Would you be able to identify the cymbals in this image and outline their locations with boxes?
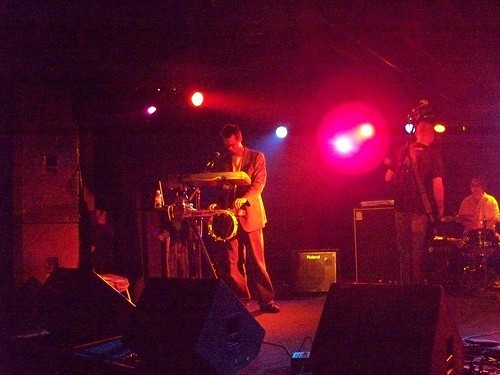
[207,209,241,242]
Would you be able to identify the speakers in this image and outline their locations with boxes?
[36,267,136,344]
[121,277,265,374]
[297,253,338,292]
[353,209,404,283]
[309,280,440,373]
[0,135,77,222]
[0,222,78,288]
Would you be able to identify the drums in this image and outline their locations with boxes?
[467,228,498,257]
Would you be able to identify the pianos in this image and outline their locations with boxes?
[167,171,252,277]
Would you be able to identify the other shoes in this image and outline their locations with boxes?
[490,278,500,289]
[259,302,280,312]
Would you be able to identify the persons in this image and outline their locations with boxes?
[207,124,280,313]
[458,174,500,288]
[384,121,445,281]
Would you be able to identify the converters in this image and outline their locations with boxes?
[290,352,311,370]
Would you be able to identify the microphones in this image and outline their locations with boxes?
[411,100,429,112]
[206,151,220,167]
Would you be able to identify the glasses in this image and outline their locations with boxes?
[223,143,238,149]
